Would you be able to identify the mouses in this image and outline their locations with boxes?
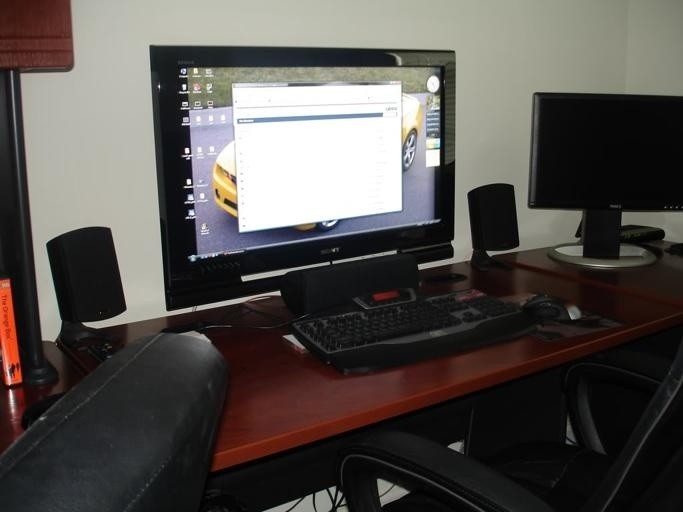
[526,293,581,323]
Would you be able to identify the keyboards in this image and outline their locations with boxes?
[292,287,537,374]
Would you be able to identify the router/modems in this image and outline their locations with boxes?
[620,224,666,245]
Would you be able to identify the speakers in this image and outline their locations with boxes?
[468,184,519,272]
[46,227,126,364]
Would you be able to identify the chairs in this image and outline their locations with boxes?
[0,328,230,512]
[336,345,682,512]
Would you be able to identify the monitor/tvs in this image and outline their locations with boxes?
[149,45,455,319]
[527,93,683,269]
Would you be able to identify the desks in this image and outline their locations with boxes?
[2,232,681,467]
[490,226,682,310]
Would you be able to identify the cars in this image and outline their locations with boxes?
[209,90,423,235]
